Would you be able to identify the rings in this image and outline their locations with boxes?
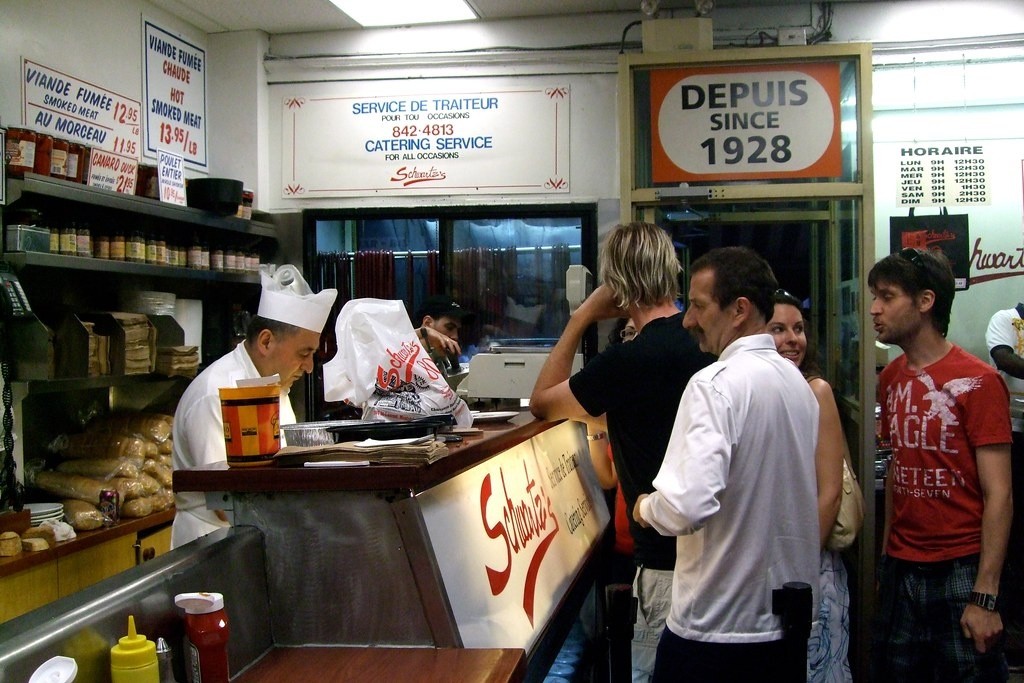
[446,339,449,342]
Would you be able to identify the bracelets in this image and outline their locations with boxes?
[586,431,606,441]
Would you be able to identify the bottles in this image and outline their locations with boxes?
[110,616,174,683]
[174,592,229,683]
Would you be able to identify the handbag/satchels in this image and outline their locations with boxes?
[808,377,865,550]
[320,297,474,434]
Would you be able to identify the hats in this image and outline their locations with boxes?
[257,264,338,332]
[419,295,472,318]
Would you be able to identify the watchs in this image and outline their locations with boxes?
[967,590,998,613]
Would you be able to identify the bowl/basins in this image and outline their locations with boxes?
[281,419,385,448]
[187,178,243,216]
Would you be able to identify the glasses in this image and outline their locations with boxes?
[900,248,927,283]
[774,288,794,296]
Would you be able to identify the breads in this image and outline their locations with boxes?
[0,409,175,558]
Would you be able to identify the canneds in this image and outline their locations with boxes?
[4,124,92,185]
[134,163,160,200]
[236,190,254,221]
[45,221,260,272]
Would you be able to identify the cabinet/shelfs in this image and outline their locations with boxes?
[0,171,281,494]
[140,526,172,564]
[0,530,139,625]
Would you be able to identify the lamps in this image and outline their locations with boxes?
[641,0,713,52]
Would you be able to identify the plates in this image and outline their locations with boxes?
[9,503,66,527]
[472,411,519,422]
[325,420,445,443]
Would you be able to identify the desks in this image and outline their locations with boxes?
[228,644,529,683]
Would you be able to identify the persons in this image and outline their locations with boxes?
[587,316,638,683]
[986,303,1023,672]
[481,296,545,339]
[171,264,338,552]
[766,287,855,683]
[868,248,1012,683]
[414,296,472,365]
[632,248,820,683]
[530,220,719,683]
